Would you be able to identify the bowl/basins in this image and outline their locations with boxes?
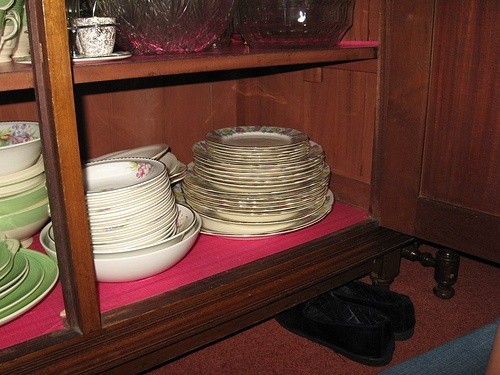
[90,0,237,55]
[234,1,355,51]
[38,202,203,283]
[0,182,49,251]
[0,122,41,174]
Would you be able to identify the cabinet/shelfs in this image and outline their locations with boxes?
[0,0,500,375]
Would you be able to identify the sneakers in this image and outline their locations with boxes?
[332,280,415,341]
[275,293,394,366]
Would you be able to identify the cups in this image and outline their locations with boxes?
[66,1,116,56]
[0,0,30,63]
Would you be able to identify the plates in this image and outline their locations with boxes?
[79,127,334,252]
[0,154,47,200]
[71,51,133,64]
[0,234,59,328]
